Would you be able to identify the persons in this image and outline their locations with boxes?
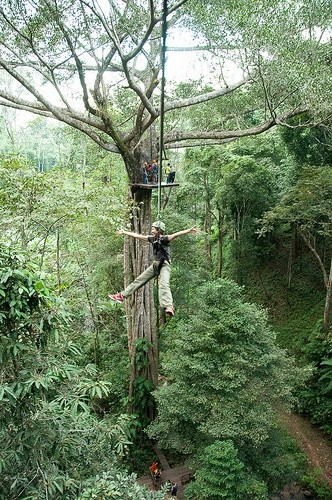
[152,469,178,498]
[141,159,175,185]
[107,221,201,317]
